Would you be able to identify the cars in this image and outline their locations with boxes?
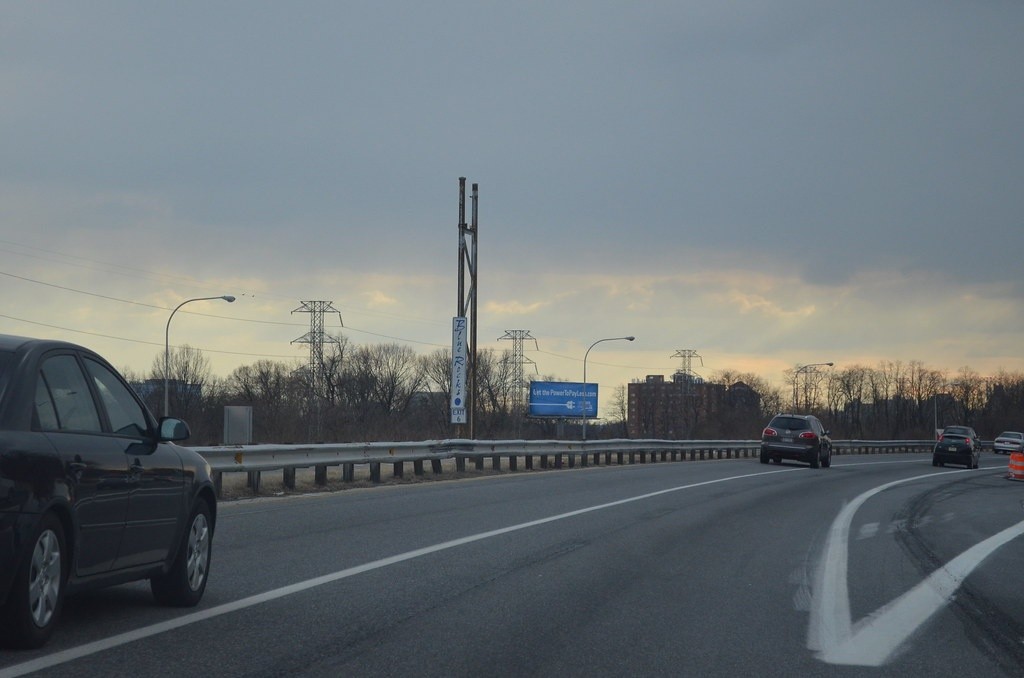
[994,430,1024,454]
[0,334,217,652]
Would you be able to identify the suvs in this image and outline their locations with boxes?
[933,433,976,469]
[944,425,981,454]
[761,412,832,469]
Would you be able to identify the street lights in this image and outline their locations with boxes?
[164,296,236,416]
[935,383,960,441]
[792,363,833,413]
[583,337,635,440]
